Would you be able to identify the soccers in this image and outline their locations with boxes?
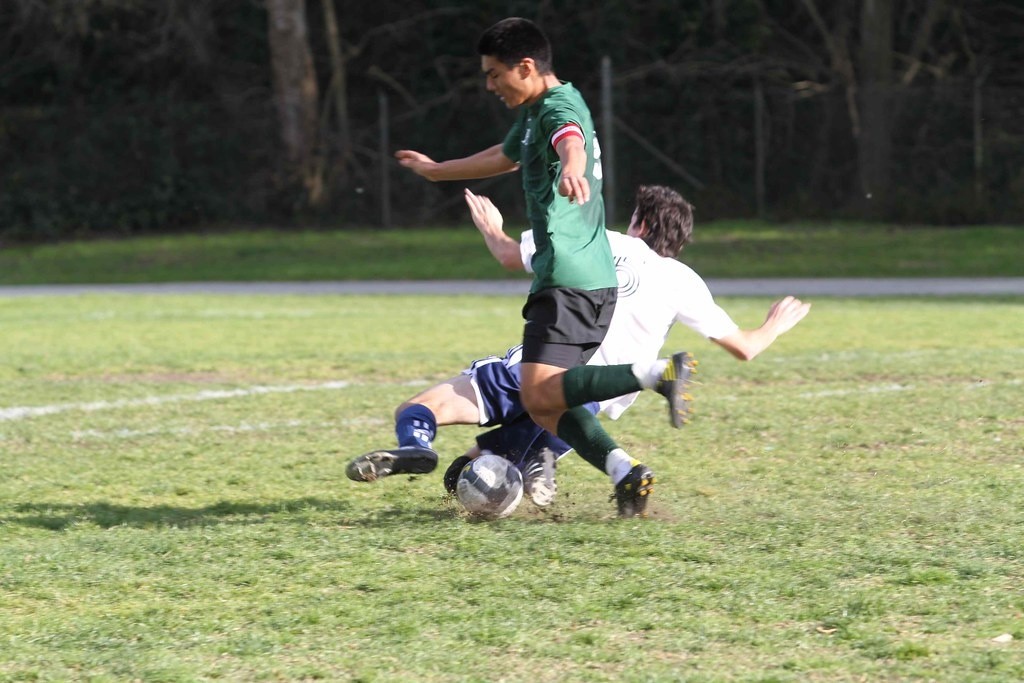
[456,453,525,522]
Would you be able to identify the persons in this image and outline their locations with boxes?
[344,187,811,505]
[394,17,700,518]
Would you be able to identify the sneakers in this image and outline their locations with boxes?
[608,460,656,518]
[346,445,438,483]
[522,447,558,506]
[654,352,699,430]
